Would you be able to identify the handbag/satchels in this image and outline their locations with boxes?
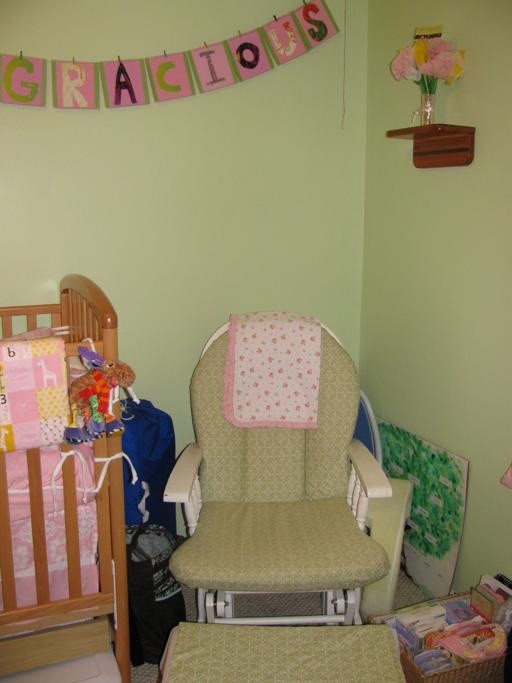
[120,397,177,540]
[107,521,189,667]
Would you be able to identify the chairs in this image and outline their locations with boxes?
[162,312,393,629]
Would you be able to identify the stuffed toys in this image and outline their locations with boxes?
[64,346,136,444]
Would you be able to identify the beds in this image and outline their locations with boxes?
[1,271,132,683]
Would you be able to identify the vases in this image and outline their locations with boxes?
[421,92,435,126]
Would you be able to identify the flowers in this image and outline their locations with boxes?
[390,36,466,94]
